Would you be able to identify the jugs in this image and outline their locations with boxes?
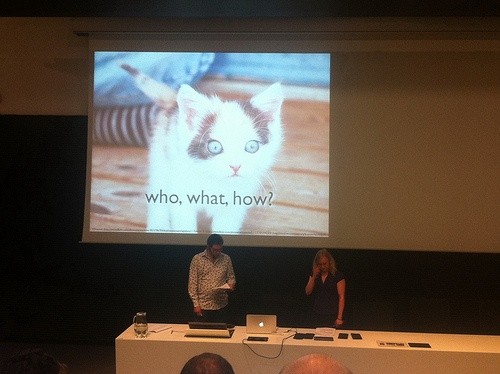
[132,312,149,337]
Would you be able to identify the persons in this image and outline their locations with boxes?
[179,353,234,374]
[188,234,236,323]
[304,249,345,329]
[278,353,352,374]
[1,349,69,374]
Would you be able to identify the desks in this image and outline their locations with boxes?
[115,322,500,374]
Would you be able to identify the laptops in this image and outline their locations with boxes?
[246,314,279,334]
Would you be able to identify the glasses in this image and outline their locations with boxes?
[213,249,223,252]
